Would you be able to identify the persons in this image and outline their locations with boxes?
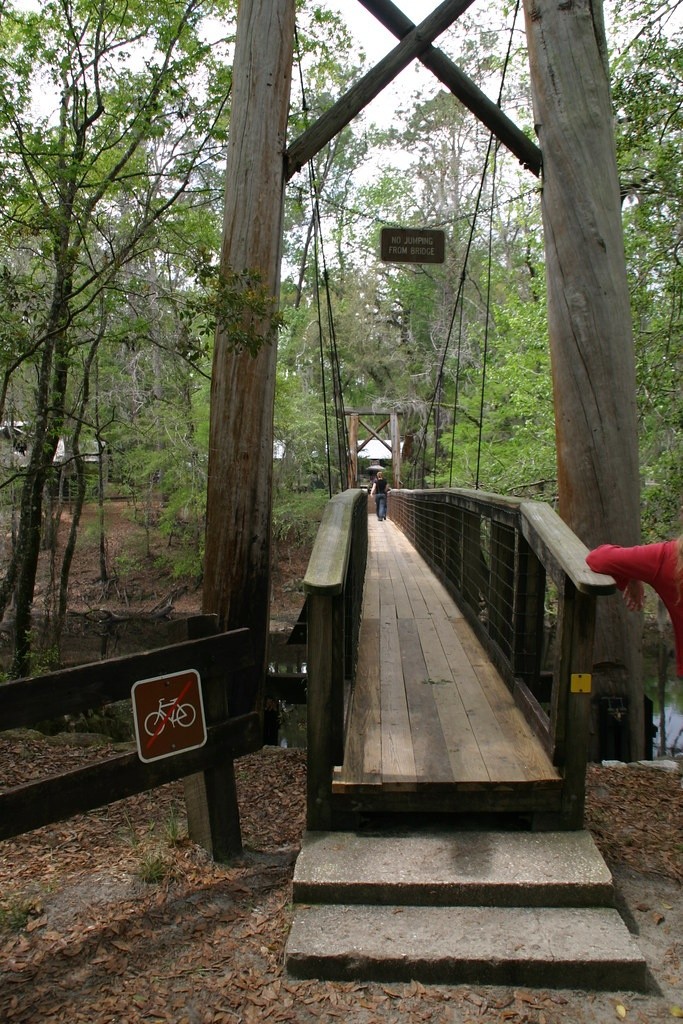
[370,471,388,521]
[585,534,683,678]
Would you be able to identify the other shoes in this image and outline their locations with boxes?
[378,517,383,521]
[383,515,387,520]
